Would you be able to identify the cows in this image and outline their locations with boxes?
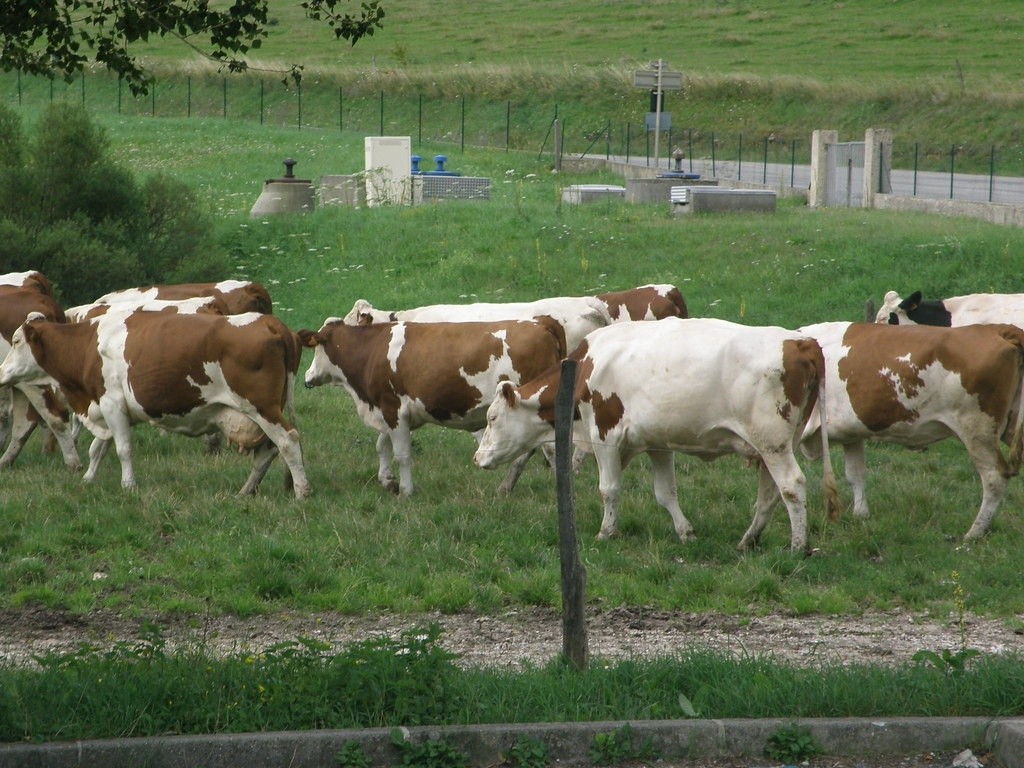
[1,268,1024,559]
